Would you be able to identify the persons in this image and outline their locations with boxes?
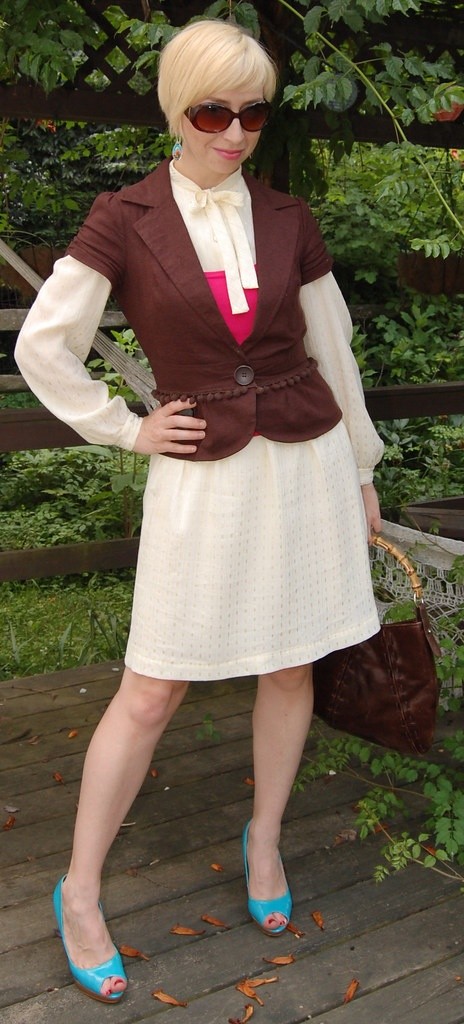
[14,20,385,1003]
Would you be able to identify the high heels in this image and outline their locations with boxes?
[53,875,127,1004]
[241,819,292,937]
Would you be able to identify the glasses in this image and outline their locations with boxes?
[187,97,275,134]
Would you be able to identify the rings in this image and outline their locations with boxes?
[373,528,382,534]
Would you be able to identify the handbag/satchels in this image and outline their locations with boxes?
[311,533,443,754]
